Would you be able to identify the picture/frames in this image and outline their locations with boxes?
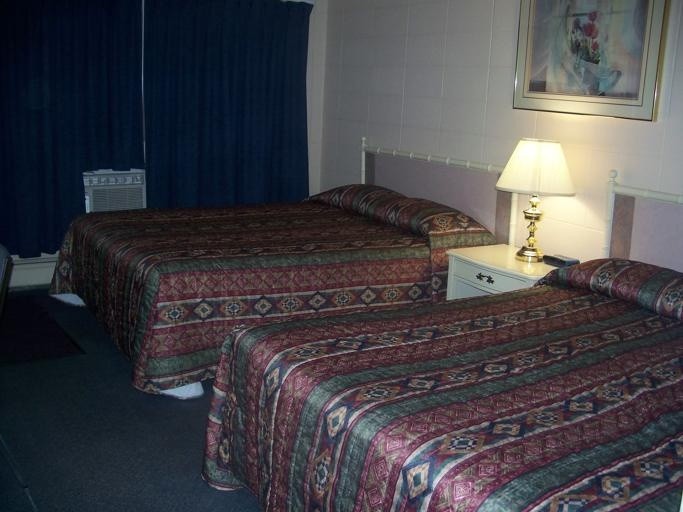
[511,0,672,124]
